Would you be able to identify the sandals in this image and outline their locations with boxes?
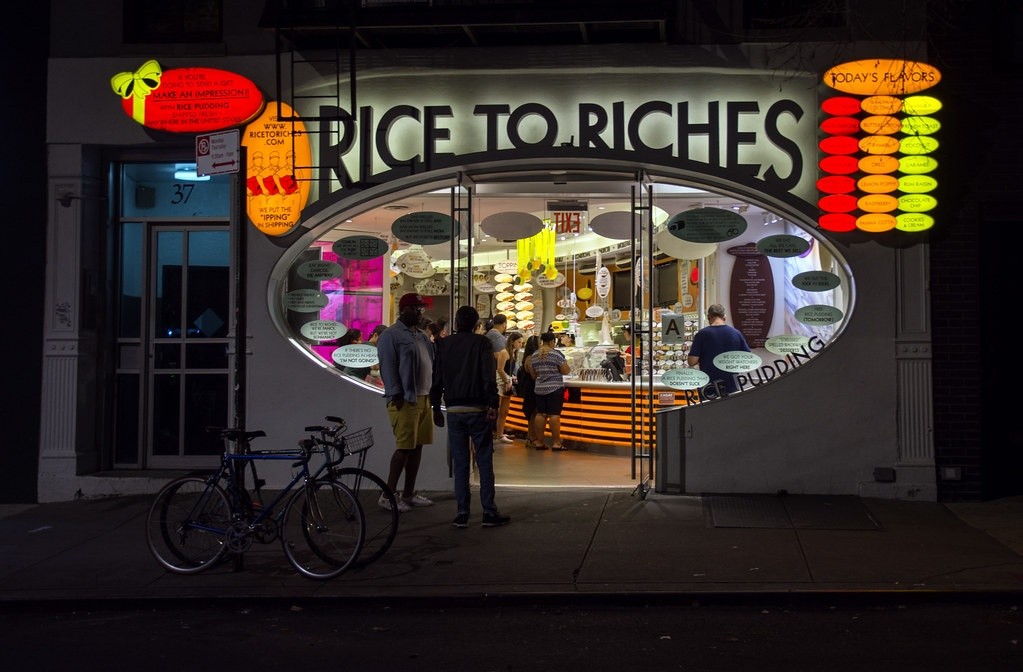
[525,438,537,446]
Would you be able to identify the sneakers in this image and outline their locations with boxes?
[378,491,413,513]
[400,493,433,507]
[481,513,511,526]
[452,513,469,527]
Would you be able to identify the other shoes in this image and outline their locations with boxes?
[503,434,515,438]
[497,436,513,443]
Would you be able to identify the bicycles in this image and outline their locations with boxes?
[145,413,401,580]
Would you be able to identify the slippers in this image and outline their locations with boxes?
[536,444,549,450]
[552,445,568,451]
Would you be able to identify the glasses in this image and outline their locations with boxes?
[415,307,425,314]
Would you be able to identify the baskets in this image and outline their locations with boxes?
[338,427,373,457]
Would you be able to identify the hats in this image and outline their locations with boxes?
[621,323,640,330]
[399,293,429,307]
[560,333,574,339]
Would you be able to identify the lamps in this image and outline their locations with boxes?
[764,213,783,226]
[174,164,210,181]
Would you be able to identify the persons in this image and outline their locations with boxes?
[429,306,511,528]
[620,321,641,382]
[686,304,752,402]
[333,314,575,452]
[377,292,435,513]
